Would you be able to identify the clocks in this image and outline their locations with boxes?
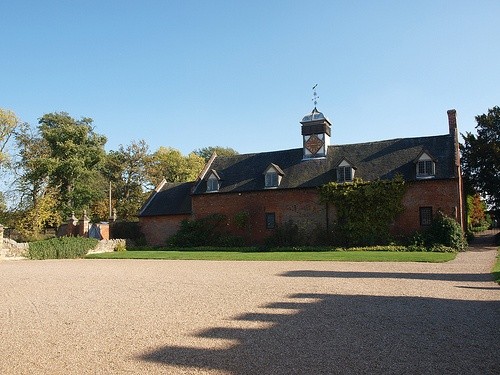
[304,134,324,157]
